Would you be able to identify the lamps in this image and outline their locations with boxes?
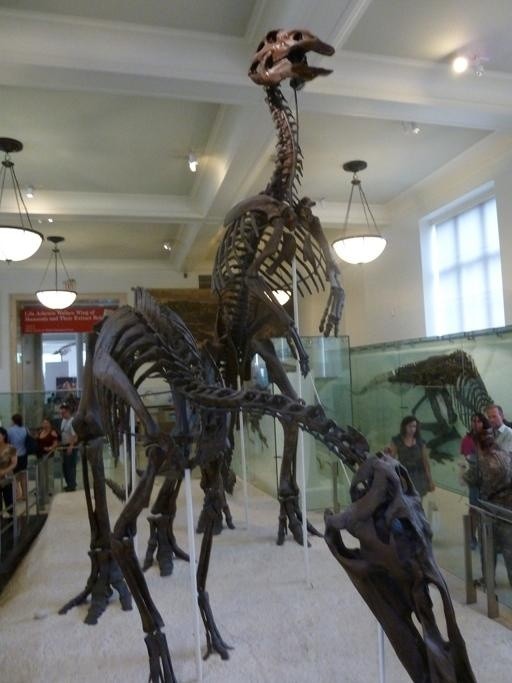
[330,159,388,266]
[261,267,292,306]
[1,136,45,263]
[26,184,33,198]
[185,150,199,174]
[451,48,488,80]
[32,236,79,312]
[400,119,423,136]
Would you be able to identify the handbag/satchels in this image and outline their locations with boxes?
[426,499,442,533]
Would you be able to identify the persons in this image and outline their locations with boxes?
[475,430,512,583]
[0,426,17,525]
[486,402,511,453]
[59,405,78,491]
[34,417,59,495]
[458,412,489,548]
[7,414,30,499]
[391,415,435,503]
